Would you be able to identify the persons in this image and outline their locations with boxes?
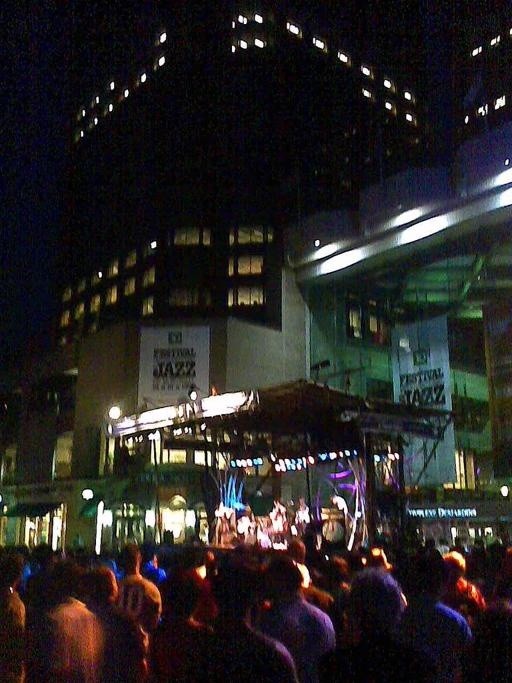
[236,503,261,546]
[212,499,236,544]
[293,497,311,535]
[2,519,510,683]
[268,498,288,546]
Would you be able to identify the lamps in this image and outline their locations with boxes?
[109,405,126,422]
[188,382,204,414]
[310,359,330,388]
